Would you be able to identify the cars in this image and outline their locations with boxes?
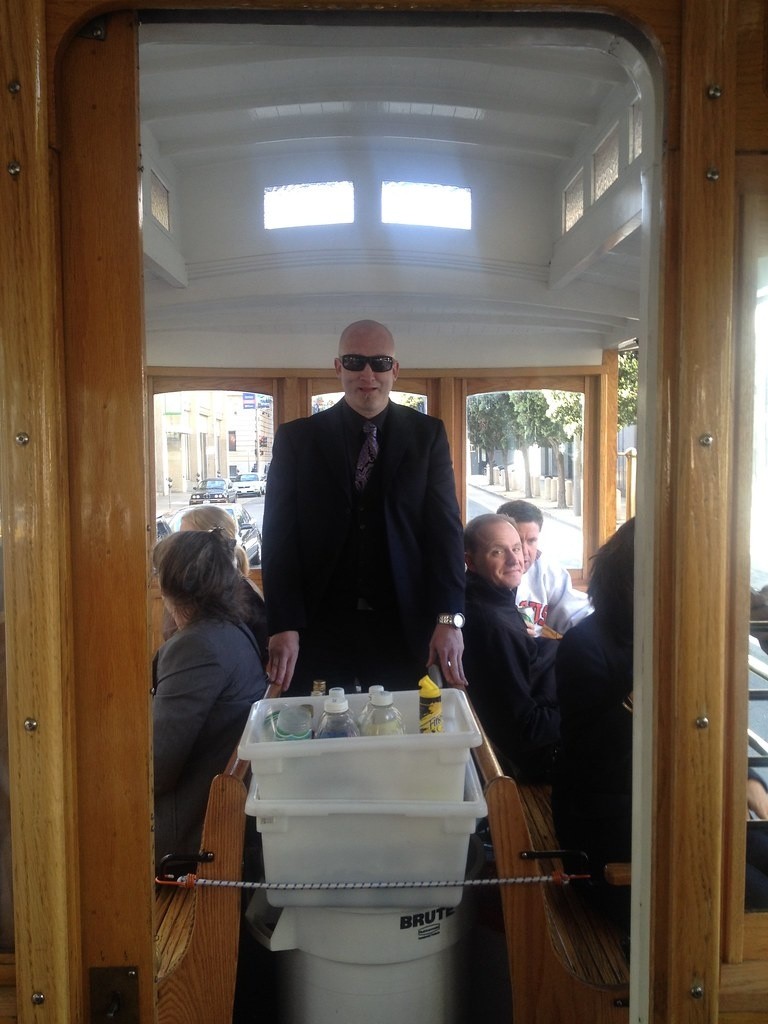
[157,502,262,568]
[189,472,268,505]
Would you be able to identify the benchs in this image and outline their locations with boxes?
[88,682,281,1024]
[444,678,631,1024]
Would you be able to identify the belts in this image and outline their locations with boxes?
[323,598,396,610]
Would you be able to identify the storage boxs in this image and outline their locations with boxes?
[237,686,488,909]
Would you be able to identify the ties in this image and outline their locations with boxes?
[354,421,380,497]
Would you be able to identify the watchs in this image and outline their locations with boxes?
[438,613,465,628]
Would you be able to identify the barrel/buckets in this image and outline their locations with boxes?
[242,835,487,1024]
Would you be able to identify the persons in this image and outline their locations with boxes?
[152,507,266,874]
[464,502,768,915]
[261,320,468,691]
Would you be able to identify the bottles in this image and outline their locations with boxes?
[268,679,407,743]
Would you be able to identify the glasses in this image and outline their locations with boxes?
[339,355,395,372]
